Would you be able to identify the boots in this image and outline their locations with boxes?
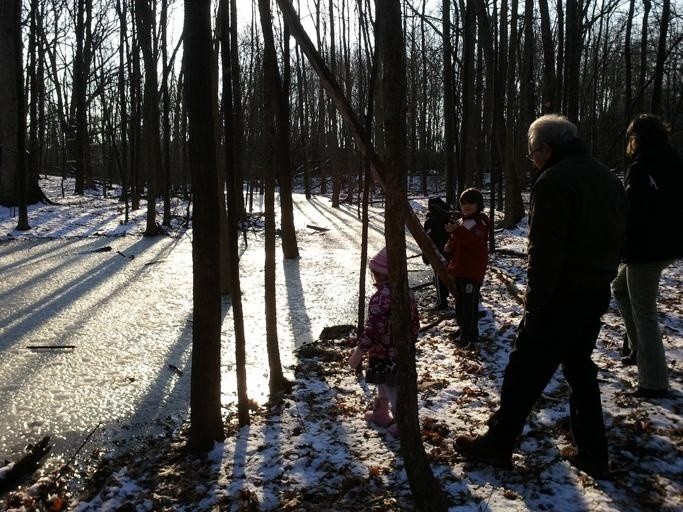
[365,397,400,437]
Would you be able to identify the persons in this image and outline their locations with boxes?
[348,246,421,429]
[613,115,683,400]
[444,187,496,347]
[456,113,630,468]
[422,197,460,312]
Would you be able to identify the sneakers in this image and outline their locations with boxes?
[621,351,636,366]
[450,328,479,346]
[453,434,512,465]
[623,387,668,399]
[429,301,448,311]
[560,446,605,473]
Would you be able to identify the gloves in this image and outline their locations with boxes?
[348,346,364,369]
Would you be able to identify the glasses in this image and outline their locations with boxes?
[525,146,543,161]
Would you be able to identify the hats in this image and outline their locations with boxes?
[369,247,388,274]
